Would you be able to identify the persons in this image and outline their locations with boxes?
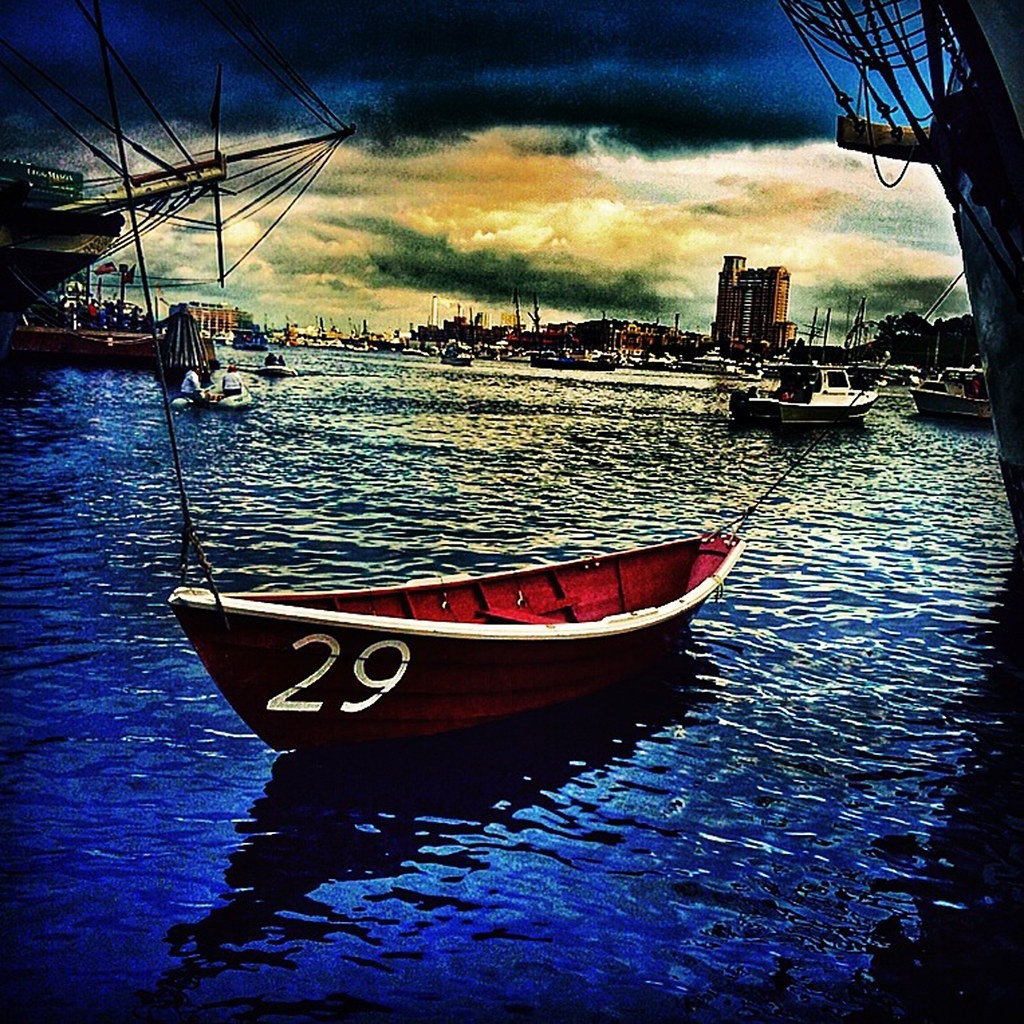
[180,351,289,408]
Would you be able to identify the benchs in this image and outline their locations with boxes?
[483,608,562,625]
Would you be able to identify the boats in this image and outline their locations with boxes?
[212,333,235,346]
[291,352,325,375]
[730,363,881,425]
[232,329,268,352]
[170,382,253,411]
[440,342,475,366]
[909,367,992,419]
[168,532,747,750]
[402,348,429,358]
[530,354,615,371]
[265,365,298,375]
[210,369,265,392]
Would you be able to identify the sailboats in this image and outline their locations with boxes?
[1,0,355,359]
